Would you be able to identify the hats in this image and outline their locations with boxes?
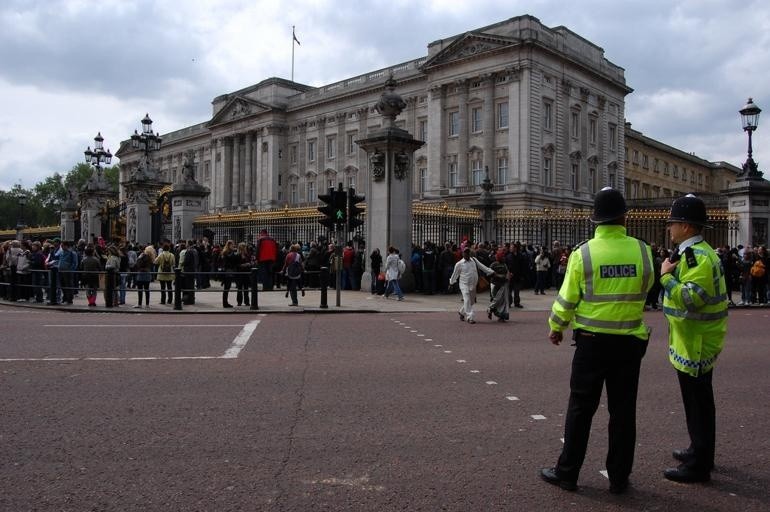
[664,193,713,230]
[588,187,629,224]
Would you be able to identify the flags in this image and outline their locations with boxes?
[293,31,300,46]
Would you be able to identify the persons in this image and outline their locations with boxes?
[370,248,382,293]
[506,244,523,308]
[487,251,513,323]
[542,188,655,494]
[382,246,405,301]
[2,230,366,306]
[448,247,496,324]
[460,240,477,303]
[636,237,769,312]
[659,194,728,484]
[410,236,577,295]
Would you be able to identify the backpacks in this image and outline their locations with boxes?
[162,253,171,272]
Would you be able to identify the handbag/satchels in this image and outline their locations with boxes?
[288,262,301,277]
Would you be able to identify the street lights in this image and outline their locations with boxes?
[735,98,767,183]
[80,131,112,191]
[127,113,162,182]
[15,192,27,226]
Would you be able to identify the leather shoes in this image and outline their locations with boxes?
[672,449,692,461]
[664,467,710,483]
[540,467,577,489]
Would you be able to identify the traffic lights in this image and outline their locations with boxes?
[316,187,335,231]
[332,191,347,225]
[346,187,366,233]
